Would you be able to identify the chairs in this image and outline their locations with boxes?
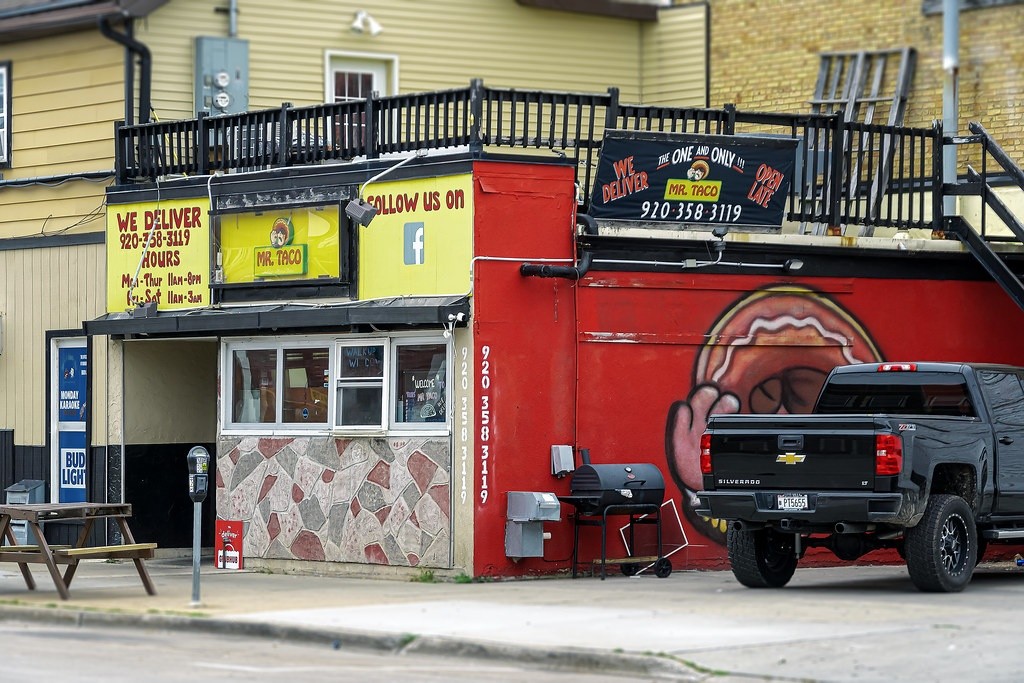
[923,396,970,416]
[843,395,885,413]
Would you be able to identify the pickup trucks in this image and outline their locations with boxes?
[695,361,1024,594]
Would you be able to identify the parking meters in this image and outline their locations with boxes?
[187,446,210,601]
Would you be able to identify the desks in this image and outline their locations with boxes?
[0,502,157,600]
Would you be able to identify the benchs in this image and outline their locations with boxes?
[57,543,157,555]
[0,544,72,551]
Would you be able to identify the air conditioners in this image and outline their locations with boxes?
[229,122,280,174]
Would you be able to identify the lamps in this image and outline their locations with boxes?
[713,226,730,252]
[129,177,160,318]
[785,259,803,271]
[350,10,367,35]
[365,16,384,37]
[344,148,428,228]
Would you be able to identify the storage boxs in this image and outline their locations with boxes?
[270,367,308,389]
